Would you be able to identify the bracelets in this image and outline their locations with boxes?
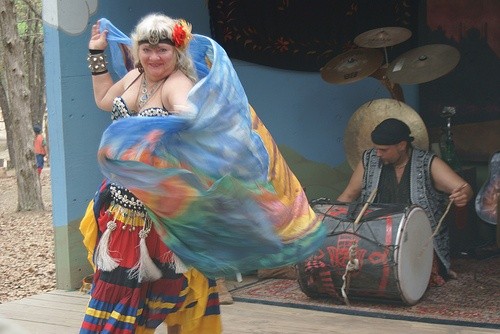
[89,49,109,75]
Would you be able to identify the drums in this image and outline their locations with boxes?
[295,196,434,306]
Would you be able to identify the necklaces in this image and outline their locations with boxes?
[396,166,405,171]
[134,71,167,112]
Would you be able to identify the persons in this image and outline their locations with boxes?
[336,117,473,283]
[79,12,328,334]
[33,126,46,176]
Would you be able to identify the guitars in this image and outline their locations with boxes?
[475,152,500,224]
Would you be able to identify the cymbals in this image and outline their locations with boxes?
[354,26,411,47]
[320,48,385,83]
[343,99,428,173]
[387,43,460,85]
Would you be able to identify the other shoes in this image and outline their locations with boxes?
[215,286,233,304]
[429,271,445,287]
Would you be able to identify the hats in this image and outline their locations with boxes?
[371,118,411,145]
